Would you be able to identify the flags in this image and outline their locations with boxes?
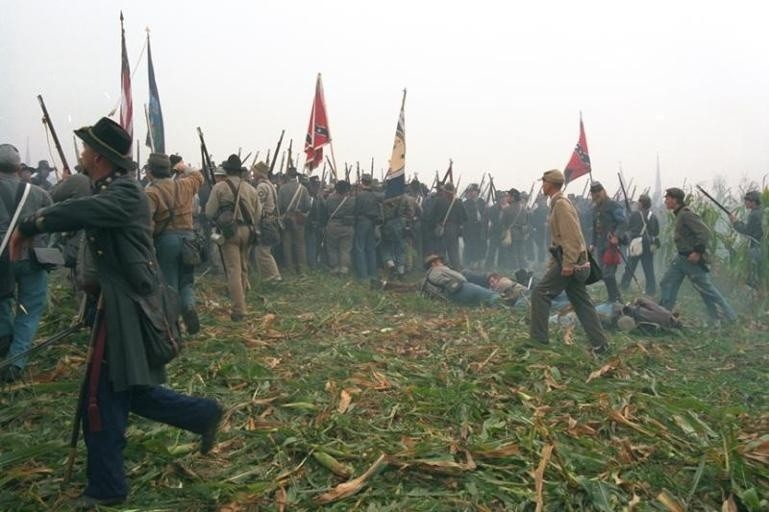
[144,39,165,155]
[118,34,134,140]
[304,75,332,171]
[562,122,591,183]
[382,100,406,199]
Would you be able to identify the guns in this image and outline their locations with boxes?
[607,233,646,297]
[527,173,651,209]
[415,173,423,198]
[135,139,142,181]
[695,184,737,220]
[430,159,497,205]
[38,94,73,177]
[63,288,104,482]
[197,127,376,189]
[72,134,81,162]
[212,221,226,274]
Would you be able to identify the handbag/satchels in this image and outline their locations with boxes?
[499,227,513,249]
[445,279,463,294]
[259,212,286,246]
[182,233,213,267]
[433,223,444,237]
[626,236,642,259]
[587,249,603,284]
[29,244,66,272]
[214,211,238,238]
[136,271,186,368]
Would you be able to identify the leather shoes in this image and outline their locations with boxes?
[4,365,20,383]
[180,300,201,336]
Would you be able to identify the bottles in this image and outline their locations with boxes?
[210,232,225,245]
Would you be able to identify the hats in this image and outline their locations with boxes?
[169,154,182,166]
[36,158,56,173]
[250,160,269,180]
[433,181,530,200]
[0,145,23,170]
[536,169,564,186]
[286,167,372,194]
[663,188,685,198]
[744,190,761,203]
[424,252,443,266]
[148,152,172,169]
[75,116,139,170]
[220,154,247,172]
[590,180,603,193]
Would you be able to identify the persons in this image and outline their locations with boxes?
[276,165,550,289]
[659,187,738,321]
[1,143,93,382]
[422,257,569,308]
[728,191,764,292]
[527,169,608,351]
[9,116,224,506]
[567,182,661,303]
[144,152,284,338]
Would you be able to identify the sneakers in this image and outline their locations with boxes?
[516,340,548,353]
[69,493,126,509]
[198,402,234,456]
